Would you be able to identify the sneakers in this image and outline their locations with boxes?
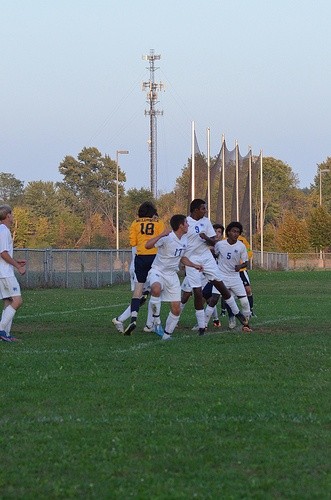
[221,307,227,317]
[112,317,124,333]
[212,319,222,327]
[0,330,16,342]
[242,317,251,332]
[228,316,236,328]
[123,321,137,336]
[192,323,208,330]
[251,309,257,318]
[144,324,155,332]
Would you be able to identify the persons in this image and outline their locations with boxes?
[112,199,257,341]
[0,206,28,342]
